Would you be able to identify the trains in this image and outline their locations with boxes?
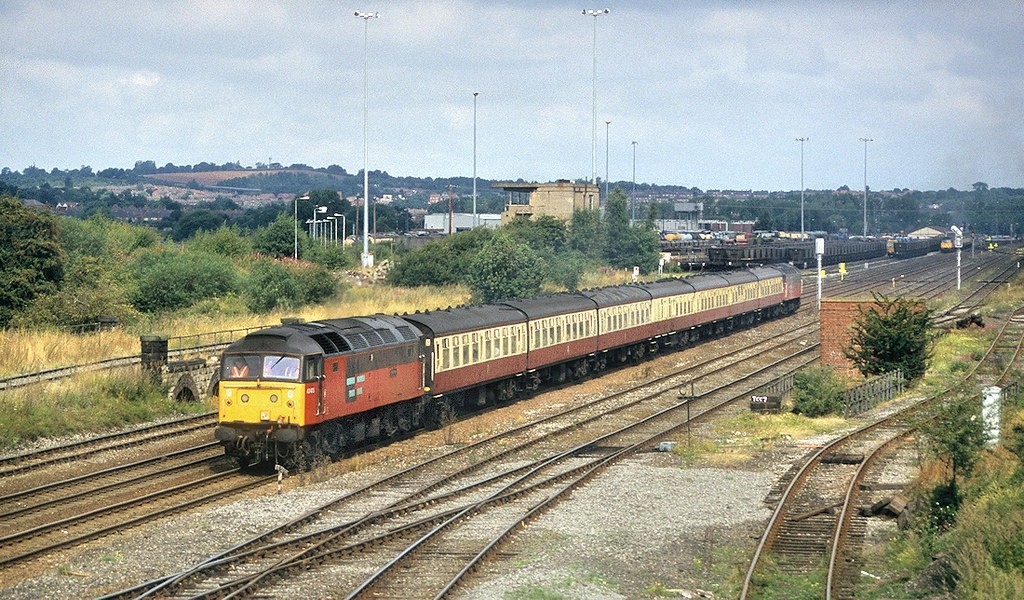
[205,261,804,476]
[653,228,978,274]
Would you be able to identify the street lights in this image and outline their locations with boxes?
[471,91,480,229]
[579,8,611,185]
[794,136,809,242]
[628,141,639,224]
[604,120,611,203]
[306,207,345,252]
[353,10,380,268]
[294,195,310,260]
[858,136,874,239]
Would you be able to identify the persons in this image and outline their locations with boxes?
[229,356,250,378]
[284,361,299,378]
